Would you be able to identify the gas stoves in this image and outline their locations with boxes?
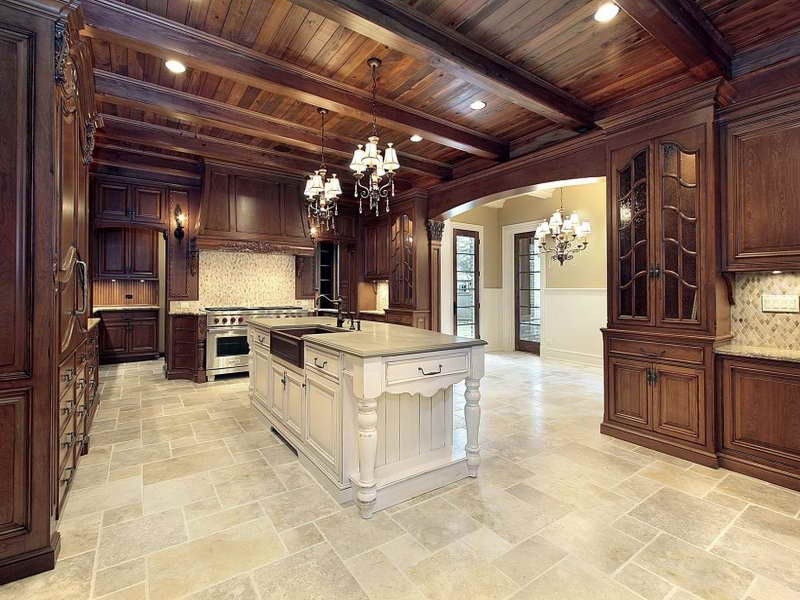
[200,305,305,327]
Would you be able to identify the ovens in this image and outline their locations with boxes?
[206,325,250,382]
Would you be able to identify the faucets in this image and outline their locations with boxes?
[315,293,348,328]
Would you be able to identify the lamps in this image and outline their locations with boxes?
[304,107,342,231]
[534,187,592,265]
[174,204,185,248]
[348,58,401,217]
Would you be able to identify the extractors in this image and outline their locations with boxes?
[192,164,316,256]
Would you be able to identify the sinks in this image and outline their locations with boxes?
[271,323,353,339]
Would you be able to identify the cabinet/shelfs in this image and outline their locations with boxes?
[596,77,800,491]
[247,325,485,519]
[56,180,198,518]
